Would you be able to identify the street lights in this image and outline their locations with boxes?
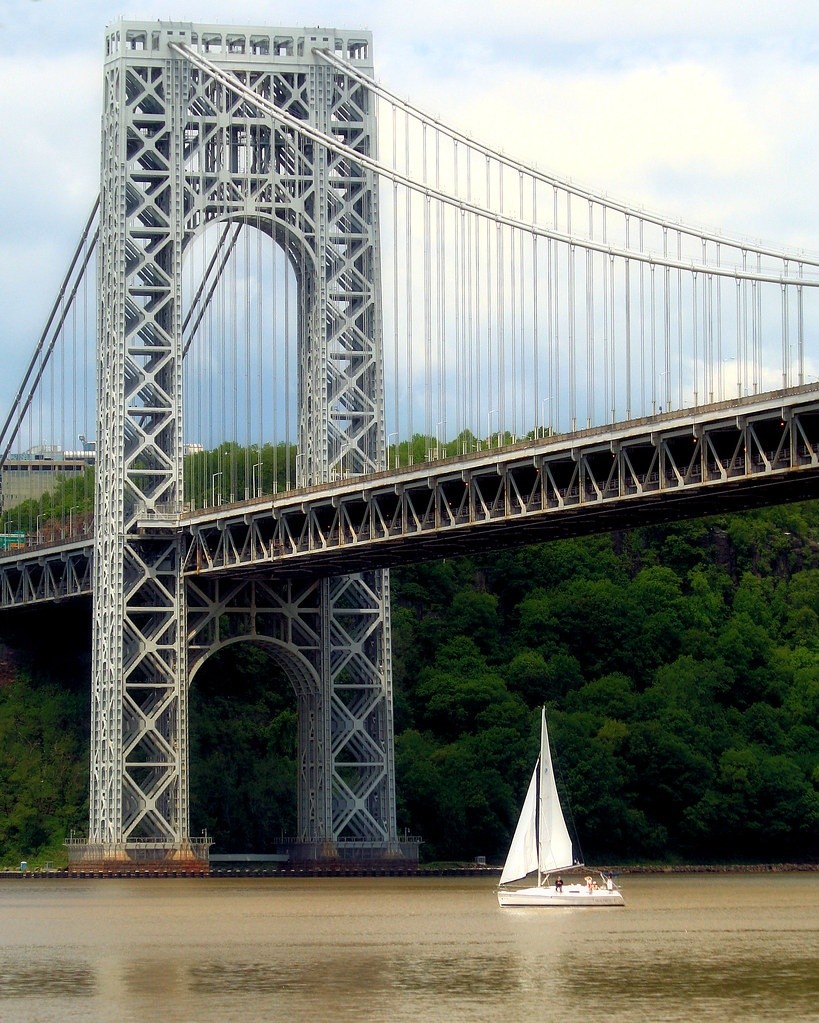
[542,396,554,438]
[4,520,13,552]
[296,454,306,489]
[252,462,263,499]
[437,421,447,461]
[488,410,499,449]
[789,341,802,387]
[212,471,223,508]
[659,371,671,413]
[388,432,398,471]
[722,355,735,401]
[340,443,351,481]
[36,513,45,546]
[69,506,79,538]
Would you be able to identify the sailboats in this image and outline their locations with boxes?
[492,704,628,906]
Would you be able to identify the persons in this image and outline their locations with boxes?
[606,875,613,894]
[590,880,600,890]
[554,876,563,895]
